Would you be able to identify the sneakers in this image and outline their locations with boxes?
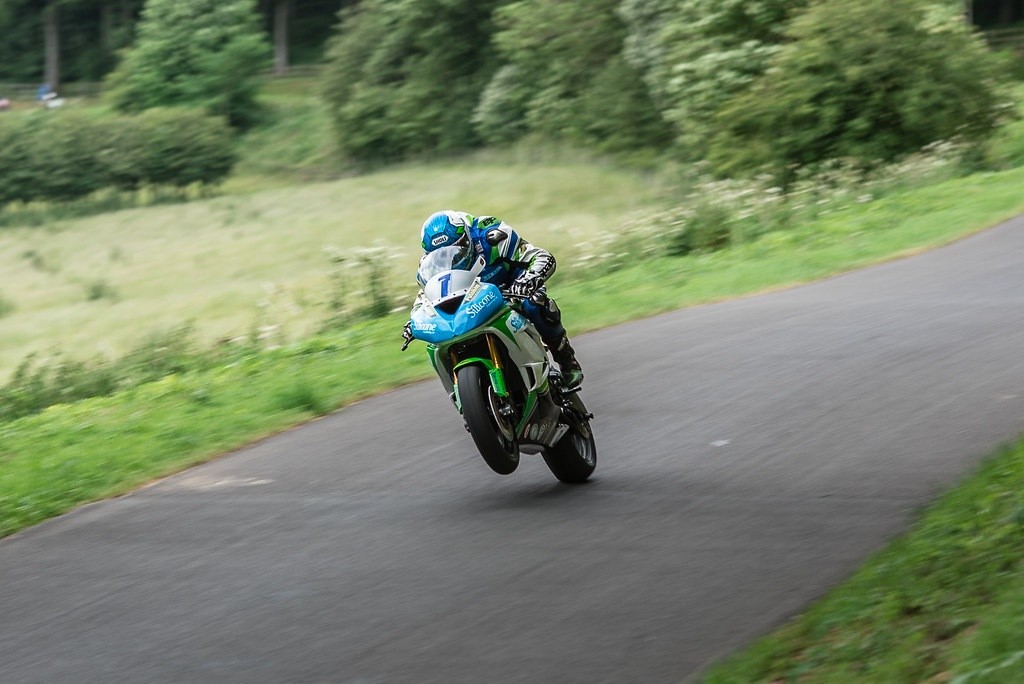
[559,356,583,388]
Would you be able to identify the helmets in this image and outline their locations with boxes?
[420,211,474,270]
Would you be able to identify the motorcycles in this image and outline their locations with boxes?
[401,243,598,484]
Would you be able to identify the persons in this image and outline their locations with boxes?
[401,210,584,414]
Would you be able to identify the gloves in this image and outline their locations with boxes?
[509,267,544,302]
[401,321,412,342]
